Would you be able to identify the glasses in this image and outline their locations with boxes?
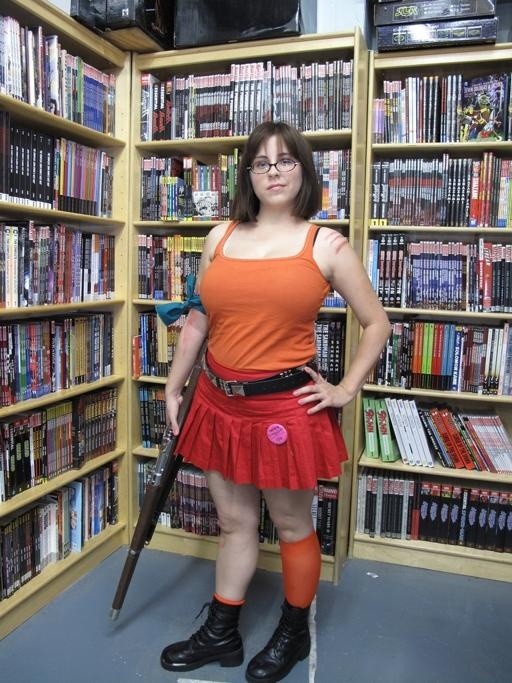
[246,159,301,174]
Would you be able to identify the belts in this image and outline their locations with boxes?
[200,342,318,397]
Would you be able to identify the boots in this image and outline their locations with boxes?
[246,598,311,683]
[162,595,244,672]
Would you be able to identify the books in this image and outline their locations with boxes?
[368,233,511,314]
[363,395,511,474]
[135,460,338,558]
[139,149,349,222]
[137,231,347,308]
[368,319,511,396]
[2,15,116,136]
[357,473,512,554]
[372,152,512,229]
[0,461,119,600]
[139,386,171,450]
[141,61,352,140]
[0,311,114,407]
[0,218,116,310]
[1,111,113,223]
[132,312,344,386]
[372,74,511,143]
[0,388,118,500]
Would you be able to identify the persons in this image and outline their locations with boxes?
[161,117,391,683]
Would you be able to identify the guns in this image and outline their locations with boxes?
[108,338,207,623]
